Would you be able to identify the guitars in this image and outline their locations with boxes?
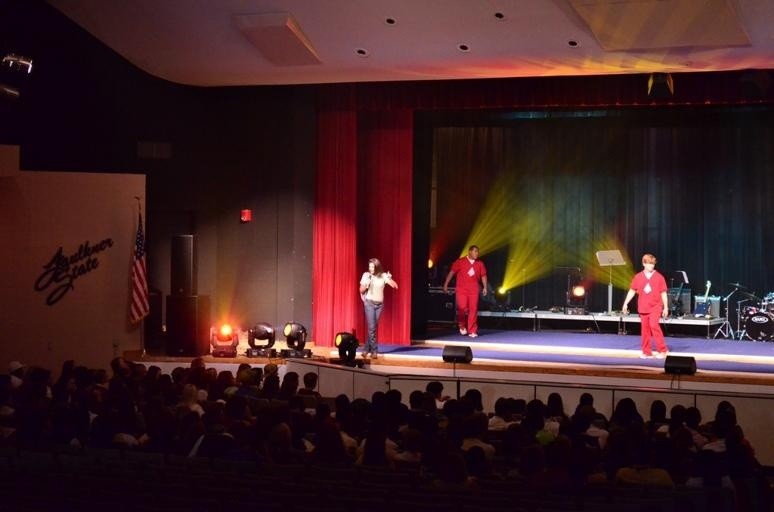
[672,282,684,315]
[693,280,712,318]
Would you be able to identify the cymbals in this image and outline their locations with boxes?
[741,292,761,301]
[728,283,747,290]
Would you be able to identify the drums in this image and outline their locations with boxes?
[744,311,774,341]
[743,306,759,319]
[764,296,774,310]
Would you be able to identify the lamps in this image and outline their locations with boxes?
[566,285,588,307]
[211,321,370,366]
[0,52,33,99]
[487,287,511,311]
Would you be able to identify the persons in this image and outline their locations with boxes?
[357,258,400,358]
[1,355,769,510]
[620,251,671,358]
[440,244,490,339]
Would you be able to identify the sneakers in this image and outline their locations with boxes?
[371,352,377,359]
[361,352,368,358]
[640,354,653,358]
[656,351,669,359]
[469,333,478,337]
[460,328,467,335]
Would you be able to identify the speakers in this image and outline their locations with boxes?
[442,346,473,363]
[665,356,696,374]
[165,296,211,356]
[170,234,197,298]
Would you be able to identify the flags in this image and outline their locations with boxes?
[125,202,151,326]
